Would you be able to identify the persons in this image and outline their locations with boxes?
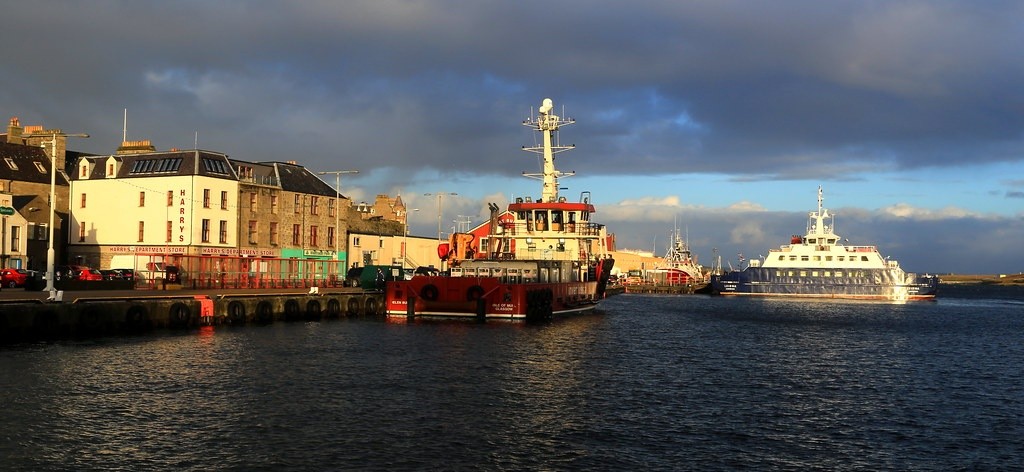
[375,268,384,292]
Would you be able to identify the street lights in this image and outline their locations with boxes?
[424,192,458,242]
[316,171,359,258]
[40,134,56,291]
[403,208,420,269]
[442,215,480,241]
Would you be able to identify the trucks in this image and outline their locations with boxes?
[346,257,448,288]
[111,255,166,285]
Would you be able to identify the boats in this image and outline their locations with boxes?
[708,184,939,301]
[653,216,703,287]
[386,79,615,320]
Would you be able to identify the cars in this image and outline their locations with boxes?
[0,265,140,292]
[608,275,619,285]
